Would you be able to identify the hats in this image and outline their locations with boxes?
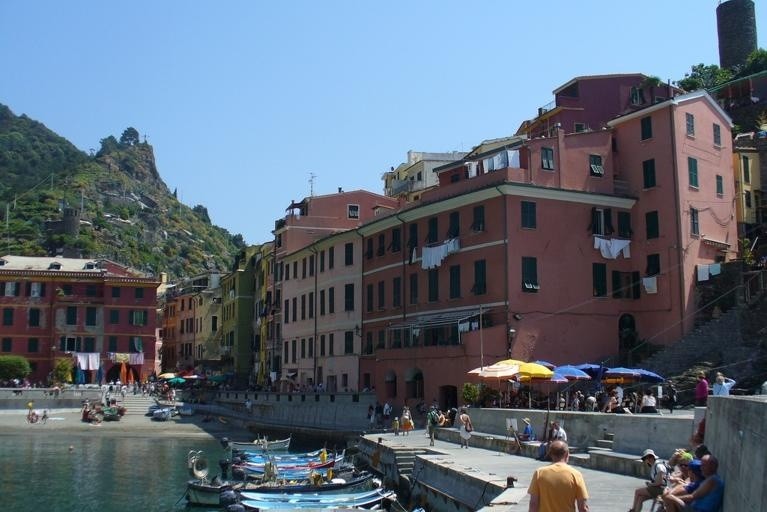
[521,418,529,426]
[641,449,659,460]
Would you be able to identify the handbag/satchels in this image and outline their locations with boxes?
[466,418,472,431]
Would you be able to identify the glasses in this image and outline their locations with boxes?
[679,463,688,468]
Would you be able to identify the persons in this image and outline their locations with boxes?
[313,359,746,511]
[1,362,311,426]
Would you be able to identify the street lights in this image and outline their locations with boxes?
[508,329,517,358]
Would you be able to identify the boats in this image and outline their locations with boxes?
[80,402,197,425]
[187,434,400,512]
